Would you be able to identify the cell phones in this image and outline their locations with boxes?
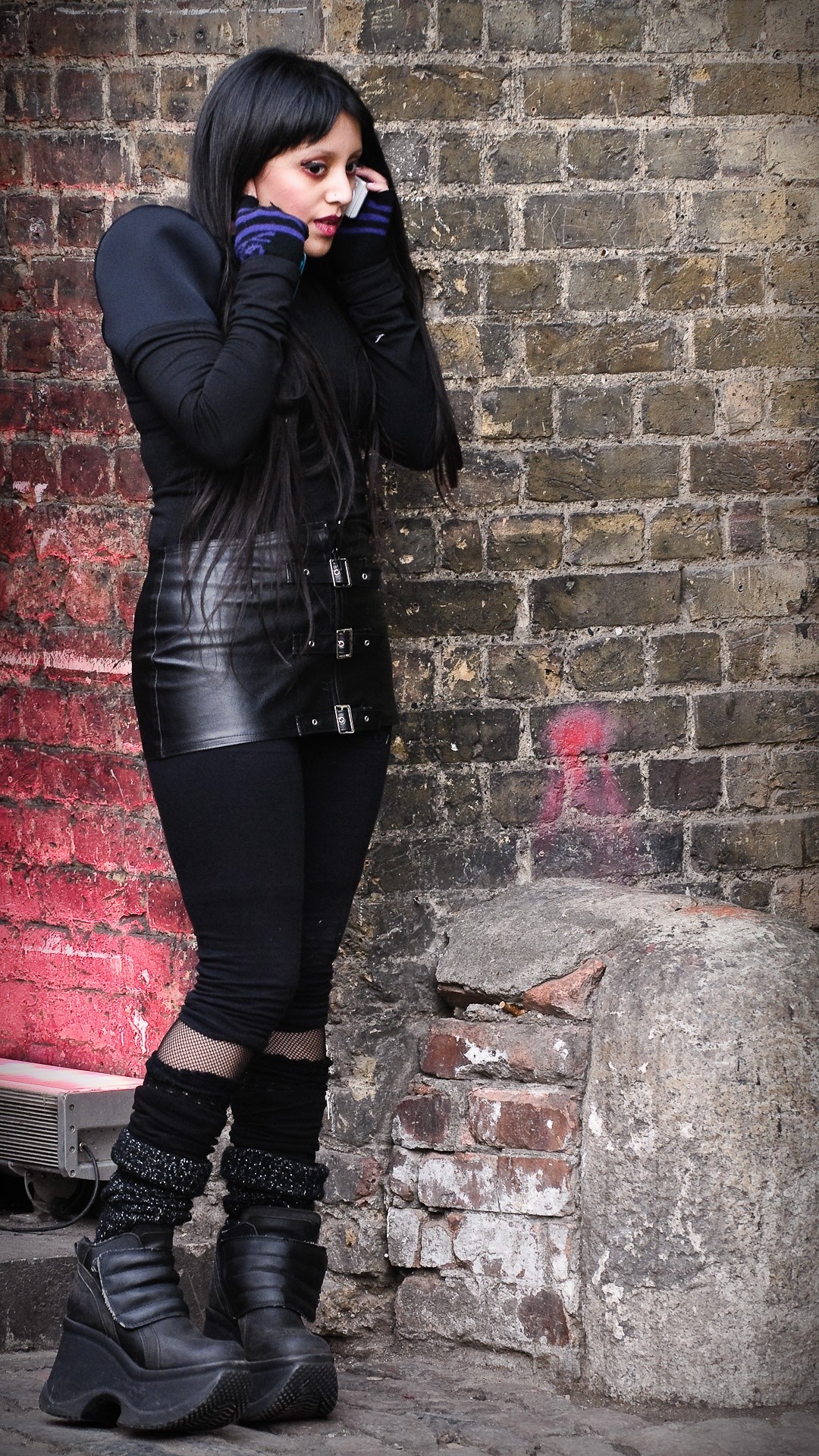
[345,174,370,217]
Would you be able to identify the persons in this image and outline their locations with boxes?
[31,44,450,1436]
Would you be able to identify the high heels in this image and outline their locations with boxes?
[200,1208,337,1429]
[38,1228,252,1441]
